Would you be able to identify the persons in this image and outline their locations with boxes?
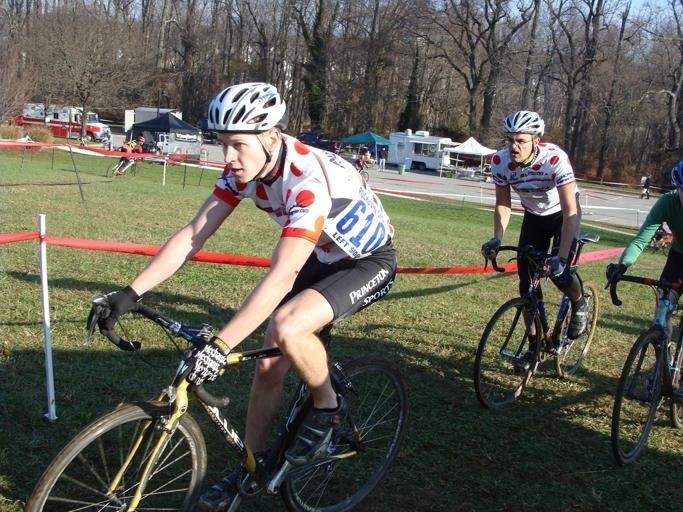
[96,80,398,510]
[639,178,650,199]
[480,110,591,374]
[16,115,25,126]
[605,161,683,395]
[330,142,350,154]
[378,147,387,172]
[112,136,145,173]
[355,154,369,171]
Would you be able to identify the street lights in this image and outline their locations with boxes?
[156,89,166,118]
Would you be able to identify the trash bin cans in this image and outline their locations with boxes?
[199,149,208,165]
[398,164,406,175]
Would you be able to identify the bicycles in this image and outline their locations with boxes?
[22,289,409,510]
[642,224,673,258]
[473,230,600,409]
[604,269,682,466]
[351,161,370,183]
[105,151,146,180]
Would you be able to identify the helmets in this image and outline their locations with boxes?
[206,80,289,133]
[503,110,546,137]
[136,136,145,143]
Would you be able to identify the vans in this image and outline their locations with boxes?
[196,111,218,144]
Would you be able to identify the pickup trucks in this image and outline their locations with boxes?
[291,131,343,157]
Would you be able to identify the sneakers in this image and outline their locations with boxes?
[197,449,274,512]
[284,392,350,467]
[514,333,541,376]
[567,292,591,340]
[643,354,674,398]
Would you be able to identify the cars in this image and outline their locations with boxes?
[116,140,162,160]
[482,163,491,173]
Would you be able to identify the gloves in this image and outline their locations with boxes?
[86,284,139,332]
[183,335,233,388]
[543,255,567,279]
[605,263,627,286]
[481,238,502,260]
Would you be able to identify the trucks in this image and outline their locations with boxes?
[16,103,111,144]
[156,131,208,158]
